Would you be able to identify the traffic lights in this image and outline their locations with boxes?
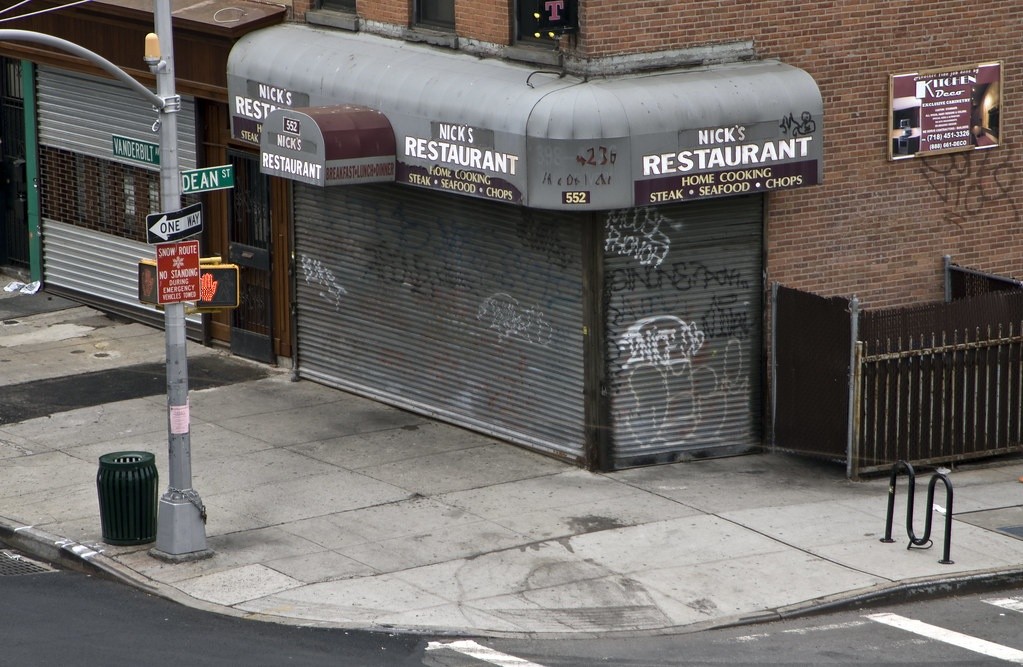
[195,266,240,310]
[137,260,159,305]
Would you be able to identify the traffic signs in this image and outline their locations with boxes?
[145,202,204,247]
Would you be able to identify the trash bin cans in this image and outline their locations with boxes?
[97,451,159,545]
